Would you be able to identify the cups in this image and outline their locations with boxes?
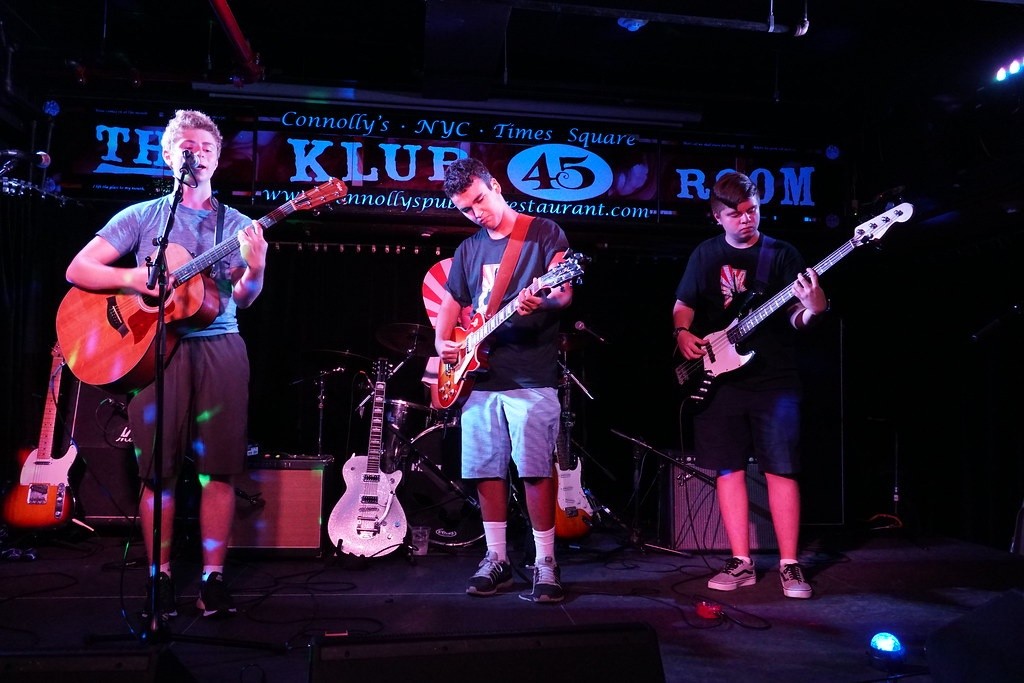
[412,526,431,555]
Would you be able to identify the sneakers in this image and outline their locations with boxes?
[708,556,756,591]
[141,570,180,618]
[464,551,514,595]
[518,556,564,603]
[195,570,238,618]
[779,561,812,599]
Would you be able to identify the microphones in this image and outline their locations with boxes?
[249,497,262,506]
[575,321,607,344]
[366,375,376,391]
[183,150,195,166]
[108,397,130,420]
[473,501,482,512]
[3,149,51,168]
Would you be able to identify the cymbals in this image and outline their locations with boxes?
[374,322,434,358]
[553,331,591,349]
[305,347,371,365]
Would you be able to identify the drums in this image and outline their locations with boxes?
[553,380,582,420]
[378,397,440,476]
[395,421,514,548]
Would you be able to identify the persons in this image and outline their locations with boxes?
[673,172,827,598]
[66,110,268,616]
[434,158,572,602]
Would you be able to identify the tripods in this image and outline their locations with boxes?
[80,164,294,655]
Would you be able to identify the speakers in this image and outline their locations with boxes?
[660,450,779,552]
[308,620,667,683]
[63,376,145,526]
[0,649,198,683]
[227,462,326,559]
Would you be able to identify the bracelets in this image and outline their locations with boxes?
[826,299,832,311]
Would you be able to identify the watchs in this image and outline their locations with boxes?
[673,327,688,338]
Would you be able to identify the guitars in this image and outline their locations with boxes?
[552,420,595,539]
[326,353,405,559]
[54,175,352,395]
[437,256,586,409]
[671,194,915,411]
[2,340,82,529]
